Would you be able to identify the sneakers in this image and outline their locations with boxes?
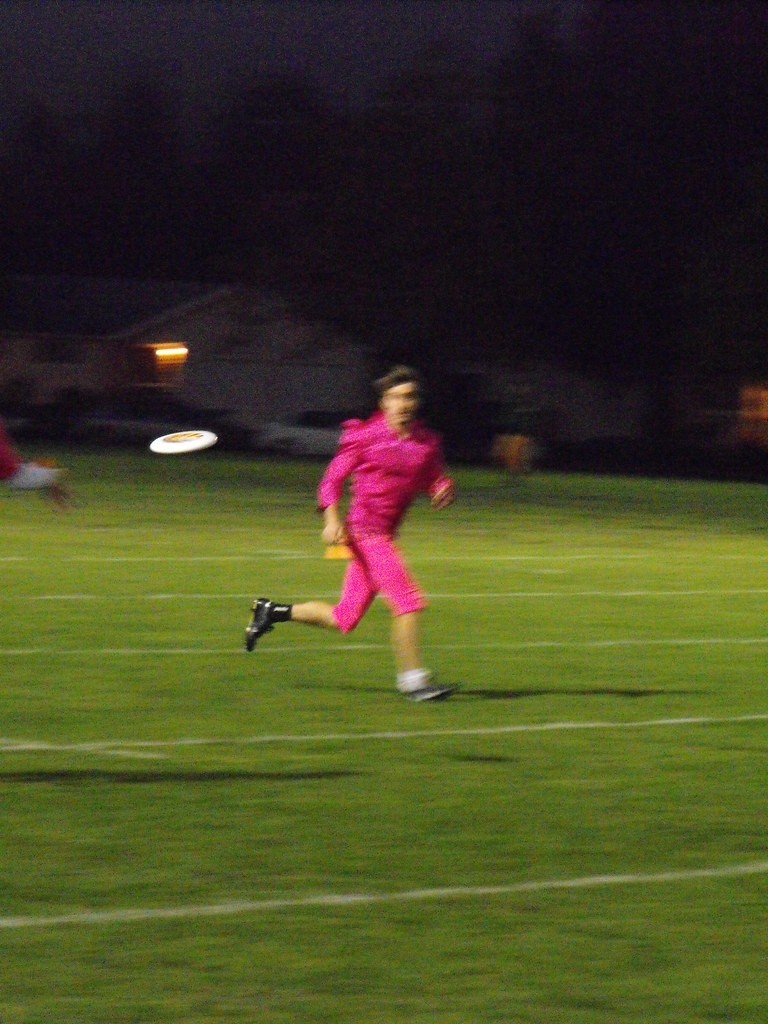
[404,681,460,703]
[244,598,274,653]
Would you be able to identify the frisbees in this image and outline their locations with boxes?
[149,430,219,456]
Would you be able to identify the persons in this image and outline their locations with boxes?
[0,428,70,506]
[244,371,457,701]
